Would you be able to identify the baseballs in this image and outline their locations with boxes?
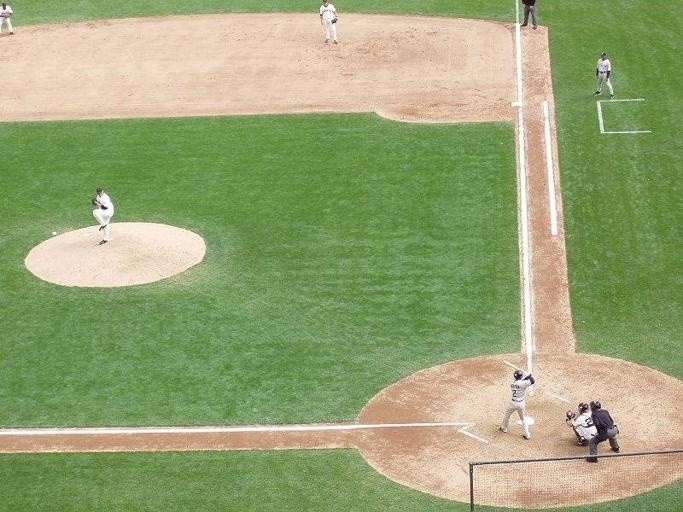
[52,231,57,235]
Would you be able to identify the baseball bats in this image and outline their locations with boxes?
[503,360,530,374]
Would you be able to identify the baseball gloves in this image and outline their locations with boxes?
[4,12,10,18]
[566,410,575,421]
[331,18,337,24]
[92,198,99,206]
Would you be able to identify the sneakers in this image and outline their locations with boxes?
[499,426,507,433]
[522,434,532,439]
[612,445,622,453]
[585,456,598,463]
[576,440,585,446]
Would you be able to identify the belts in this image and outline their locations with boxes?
[610,425,617,429]
[584,423,595,428]
[512,398,525,402]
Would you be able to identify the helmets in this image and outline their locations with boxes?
[590,400,602,409]
[514,370,523,378]
[578,402,588,409]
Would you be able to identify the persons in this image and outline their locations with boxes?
[521,0,538,30]
[0,3,14,35]
[320,0,338,44]
[92,188,114,245]
[499,369,535,440]
[566,400,621,463]
[594,52,615,100]
